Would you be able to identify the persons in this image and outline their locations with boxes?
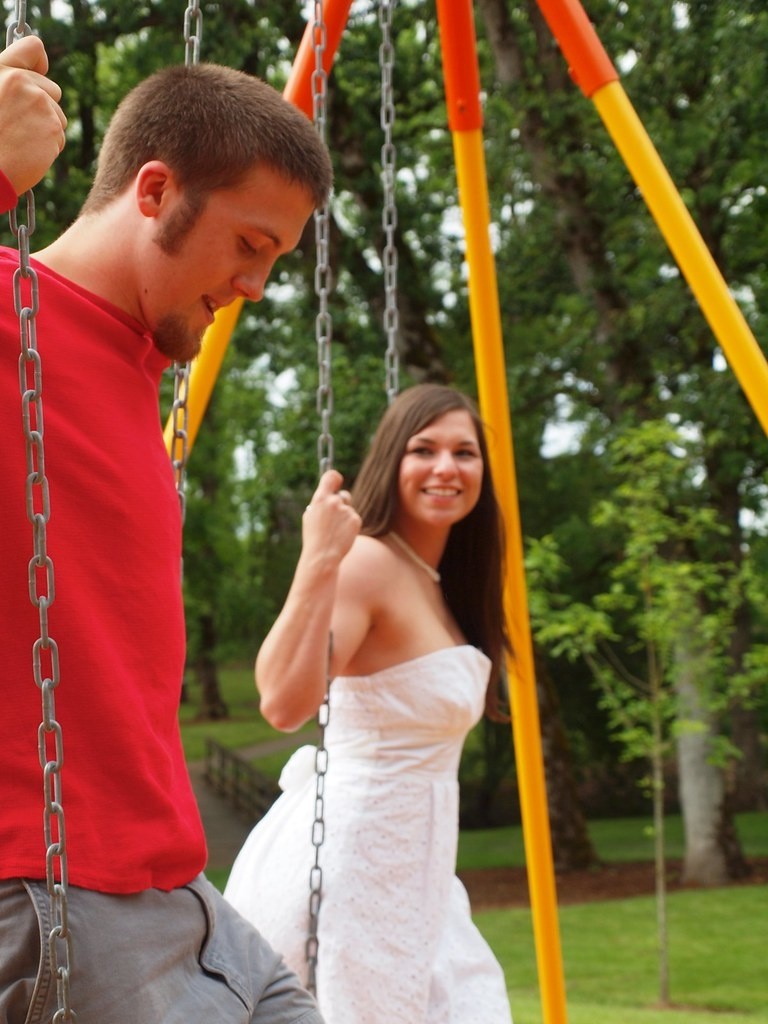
[224,385,513,1023]
[0,31,332,1022]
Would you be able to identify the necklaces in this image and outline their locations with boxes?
[389,530,440,583]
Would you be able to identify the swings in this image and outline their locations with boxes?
[0,0,401,1024]
